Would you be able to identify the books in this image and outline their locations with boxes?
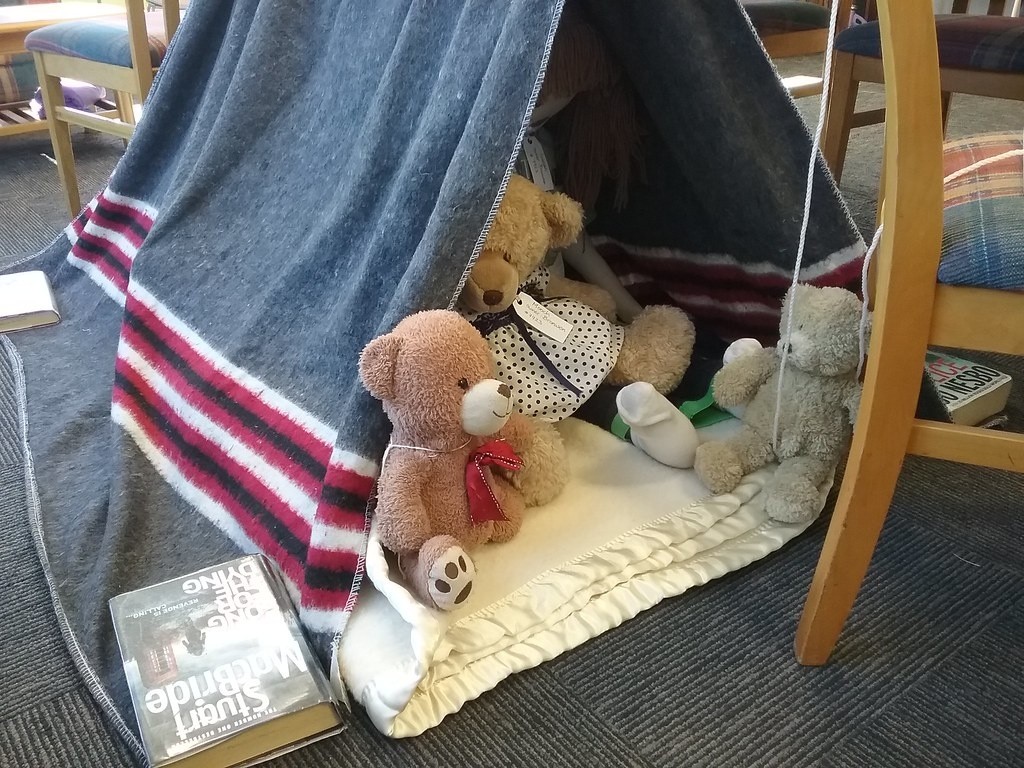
[922,350,1011,428]
[0,270,61,334]
[111,555,347,768]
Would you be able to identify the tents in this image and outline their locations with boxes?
[0,1,953,765]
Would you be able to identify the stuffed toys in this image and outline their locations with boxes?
[358,12,696,611]
[694,282,873,524]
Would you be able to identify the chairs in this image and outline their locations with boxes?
[740,0,1024,667]
[24,0,180,218]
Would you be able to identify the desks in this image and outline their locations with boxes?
[0,4,136,151]
